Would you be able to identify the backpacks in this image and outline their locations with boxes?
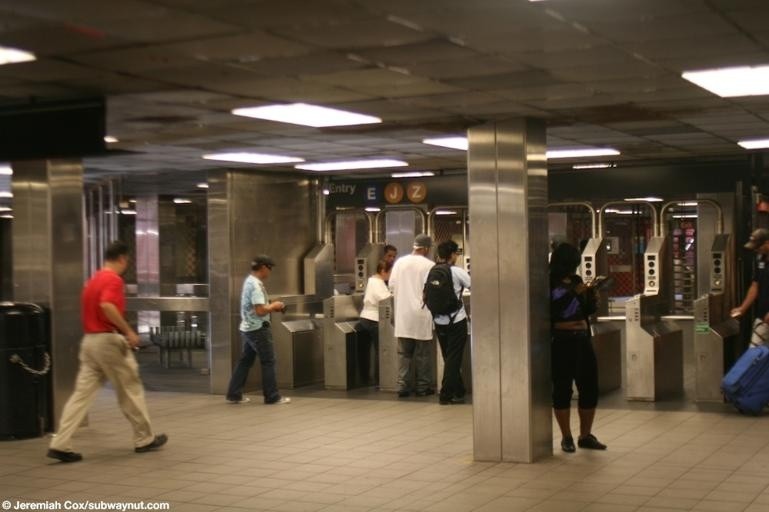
[423,261,465,313]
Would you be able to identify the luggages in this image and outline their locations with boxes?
[716,344,768,417]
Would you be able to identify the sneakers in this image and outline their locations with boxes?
[133,434,169,455]
[225,395,251,406]
[560,432,608,453]
[45,446,84,463]
[265,395,292,406]
[398,387,466,406]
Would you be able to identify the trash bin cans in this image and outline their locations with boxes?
[0,301,53,442]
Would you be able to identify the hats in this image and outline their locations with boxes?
[743,228,769,251]
[412,233,434,249]
[250,254,278,268]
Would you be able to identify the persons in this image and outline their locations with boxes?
[730,228,769,408]
[382,245,397,266]
[44,238,169,462]
[548,241,610,452]
[422,239,471,405]
[358,258,394,390]
[388,232,438,398]
[224,254,292,405]
